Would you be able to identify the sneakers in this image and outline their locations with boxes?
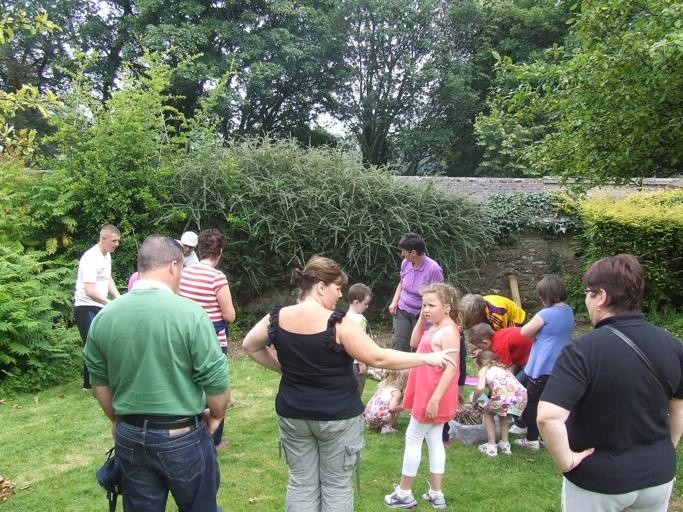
[513,438,540,451]
[509,425,528,434]
[422,490,446,508]
[382,425,399,434]
[479,444,497,457]
[499,441,511,455]
[384,490,417,508]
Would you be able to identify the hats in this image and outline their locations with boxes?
[181,231,199,247]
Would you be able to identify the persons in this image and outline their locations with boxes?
[384,284,461,509]
[388,235,444,354]
[468,322,538,388]
[169,231,200,269]
[514,276,575,451]
[536,254,682,512]
[471,350,528,456]
[242,257,459,511]
[343,284,374,399]
[81,235,231,511]
[74,224,123,391]
[175,230,236,448]
[364,368,410,433]
[458,295,529,330]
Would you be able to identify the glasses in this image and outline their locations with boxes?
[583,289,597,294]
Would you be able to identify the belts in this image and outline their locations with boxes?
[119,414,205,430]
